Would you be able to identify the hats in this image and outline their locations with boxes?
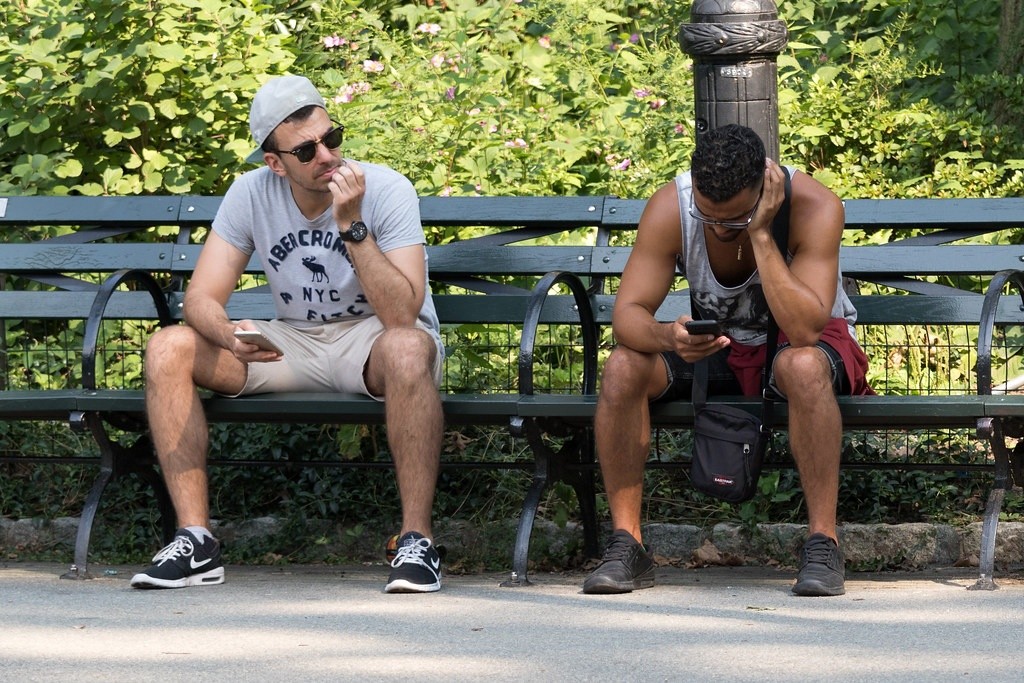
[243,75,326,164]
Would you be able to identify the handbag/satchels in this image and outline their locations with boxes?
[690,404,762,500]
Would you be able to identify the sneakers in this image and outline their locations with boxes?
[130,528,225,588]
[385,531,442,593]
[792,533,845,596]
[583,528,654,593]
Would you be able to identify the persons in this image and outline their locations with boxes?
[581,122,869,596]
[130,73,446,595]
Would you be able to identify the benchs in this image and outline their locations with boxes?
[0,194,1024,594]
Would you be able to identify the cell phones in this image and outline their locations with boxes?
[234,331,284,357]
[685,320,722,338]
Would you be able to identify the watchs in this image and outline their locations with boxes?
[338,221,367,243]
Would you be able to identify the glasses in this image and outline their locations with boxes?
[689,189,762,228]
[277,118,344,164]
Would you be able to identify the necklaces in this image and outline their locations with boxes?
[733,234,752,261]
